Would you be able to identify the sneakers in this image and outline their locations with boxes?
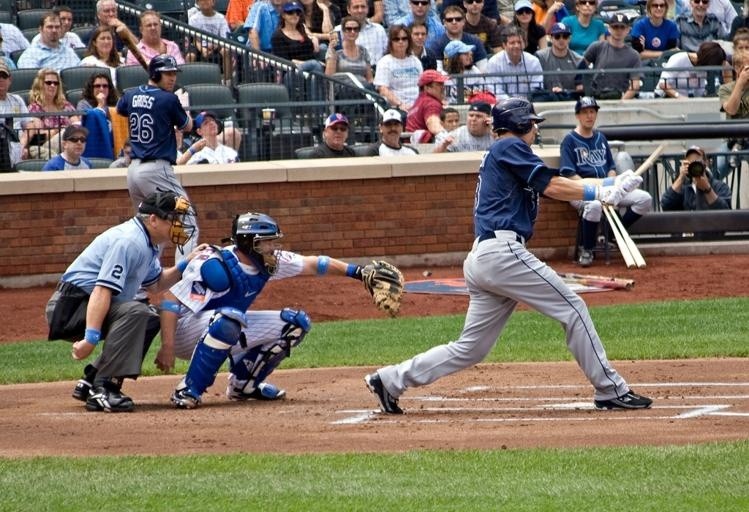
[594,390,652,409]
[595,234,617,248]
[578,250,594,267]
[170,389,197,408]
[72,376,134,413]
[227,382,285,402]
[363,371,403,413]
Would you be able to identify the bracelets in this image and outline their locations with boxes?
[83,328,101,345]
[176,257,190,274]
[602,176,617,187]
[345,261,359,277]
[581,181,597,203]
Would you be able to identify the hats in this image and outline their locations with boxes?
[63,125,89,139]
[417,70,449,87]
[575,95,600,113]
[550,22,571,34]
[609,14,629,28]
[382,109,403,125]
[0,63,11,76]
[444,39,476,58]
[196,110,216,129]
[514,0,533,11]
[469,103,489,113]
[325,114,349,128]
[685,146,705,159]
[284,3,302,12]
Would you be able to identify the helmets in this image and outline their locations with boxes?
[232,212,284,276]
[138,190,197,255]
[493,98,546,136]
[149,53,182,84]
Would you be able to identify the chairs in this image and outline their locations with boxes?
[172,62,222,93]
[123,88,144,96]
[236,83,300,129]
[65,88,85,106]
[1,1,384,70]
[7,67,49,91]
[384,0,749,101]
[9,89,31,107]
[59,66,111,91]
[181,84,236,129]
[116,65,149,92]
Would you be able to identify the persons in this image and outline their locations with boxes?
[115,53,200,301]
[154,213,406,408]
[0,0,749,171]
[661,146,732,239]
[559,97,653,267]
[43,186,212,413]
[362,98,654,412]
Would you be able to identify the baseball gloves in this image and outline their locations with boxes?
[361,261,405,315]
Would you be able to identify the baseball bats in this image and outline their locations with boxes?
[560,274,635,291]
[601,205,647,268]
[605,141,668,205]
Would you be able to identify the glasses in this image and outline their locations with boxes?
[392,37,408,42]
[343,27,359,32]
[412,2,429,5]
[446,16,464,22]
[63,138,87,143]
[45,80,59,86]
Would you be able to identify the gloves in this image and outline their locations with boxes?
[614,170,644,191]
[595,186,626,206]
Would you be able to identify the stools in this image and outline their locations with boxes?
[572,205,620,266]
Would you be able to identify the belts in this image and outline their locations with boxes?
[477,233,522,244]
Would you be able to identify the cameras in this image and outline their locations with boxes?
[688,161,704,177]
